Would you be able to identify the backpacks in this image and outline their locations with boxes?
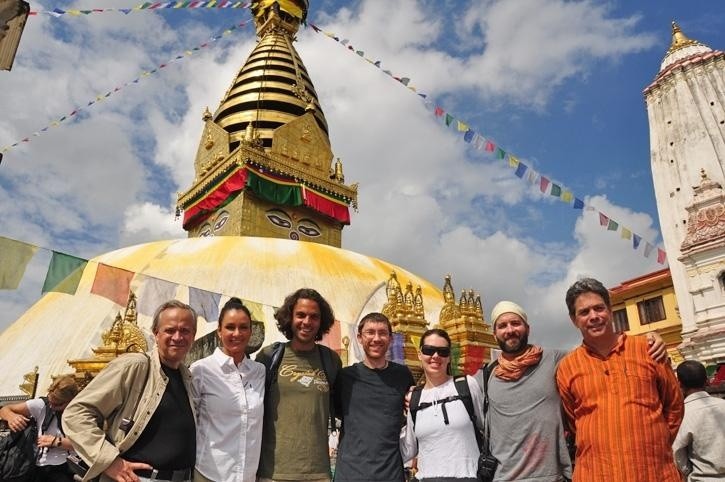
[0,396,53,481]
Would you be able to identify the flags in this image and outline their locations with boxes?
[462,341,487,377]
[134,271,180,321]
[26,0,257,19]
[188,287,223,323]
[315,320,343,355]
[447,341,461,377]
[302,16,668,266]
[384,332,403,365]
[347,323,367,363]
[408,333,422,355]
[489,347,504,365]
[39,249,88,299]
[88,260,134,312]
[241,299,267,326]
[0,235,38,293]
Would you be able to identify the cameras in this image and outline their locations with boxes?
[477,456,498,482]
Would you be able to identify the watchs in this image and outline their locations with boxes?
[55,436,64,449]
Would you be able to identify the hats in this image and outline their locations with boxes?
[491,301,527,331]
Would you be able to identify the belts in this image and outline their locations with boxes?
[135,468,194,481]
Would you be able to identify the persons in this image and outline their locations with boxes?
[552,276,688,482]
[400,299,671,482]
[0,374,80,481]
[672,359,725,482]
[185,295,269,482]
[56,299,199,481]
[329,311,419,481]
[254,288,345,481]
[396,327,490,479]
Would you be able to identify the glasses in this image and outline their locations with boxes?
[360,330,391,338]
[420,344,450,357]
